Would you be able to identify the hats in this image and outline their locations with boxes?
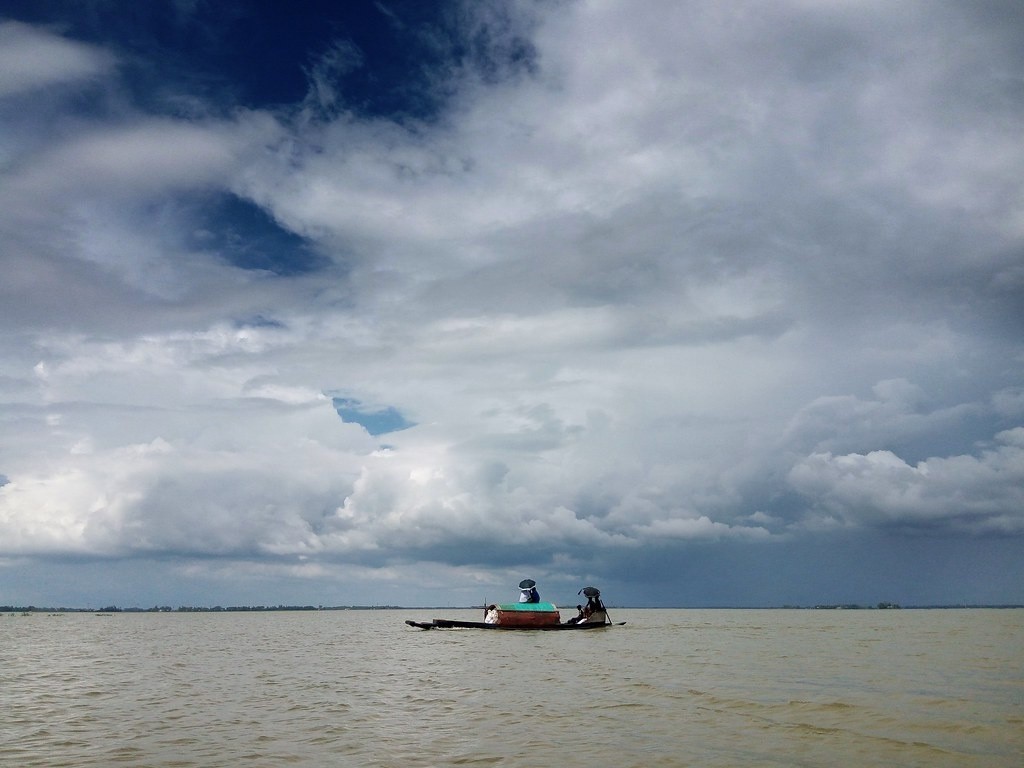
[577,605,581,608]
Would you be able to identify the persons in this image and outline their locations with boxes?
[518,587,540,603]
[568,597,601,623]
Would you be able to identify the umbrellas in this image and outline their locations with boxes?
[518,579,536,591]
[583,587,601,597]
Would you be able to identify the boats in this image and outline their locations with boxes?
[405,599,627,632]
